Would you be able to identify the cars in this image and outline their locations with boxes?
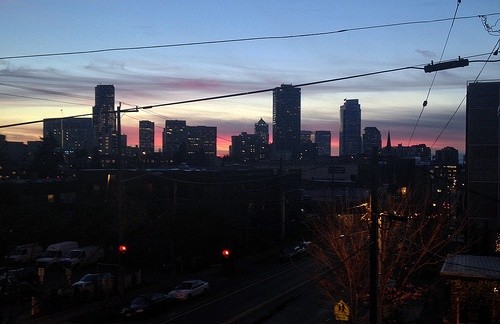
[3,220,220,317]
[281,241,313,258]
